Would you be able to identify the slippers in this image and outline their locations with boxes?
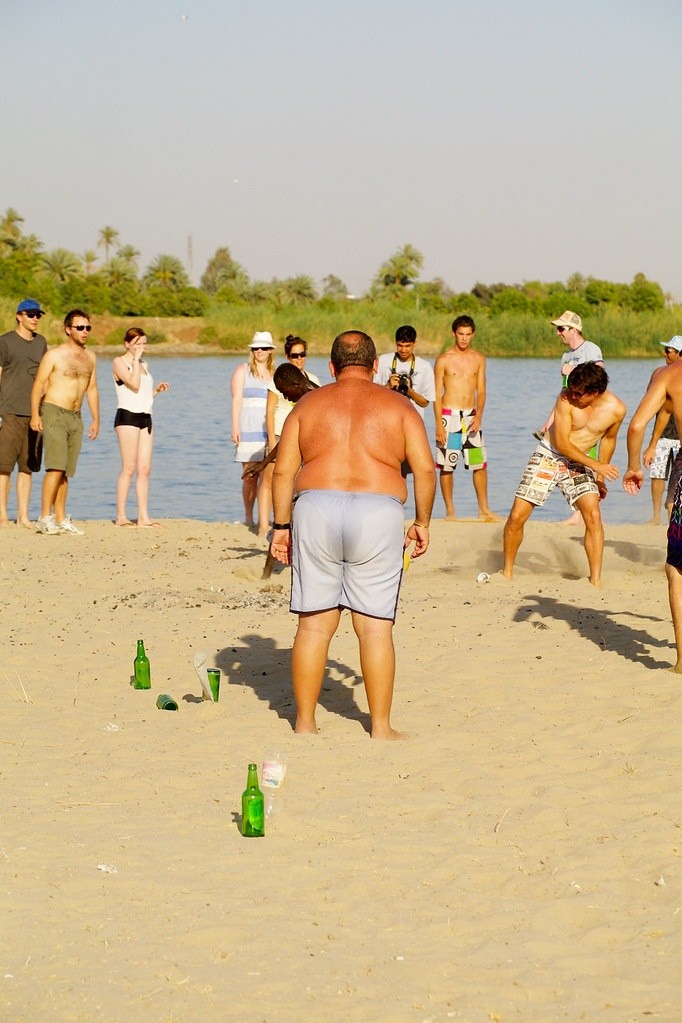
[148,522,165,529]
[120,521,135,527]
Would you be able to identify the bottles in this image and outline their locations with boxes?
[202,668,220,703]
[134,639,151,690]
[156,694,178,711]
[241,762,266,838]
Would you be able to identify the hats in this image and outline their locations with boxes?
[17,300,46,316]
[550,311,584,332]
[248,331,275,349]
[657,335,682,352]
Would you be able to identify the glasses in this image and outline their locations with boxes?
[558,326,569,332]
[22,311,41,318]
[568,391,585,396]
[251,347,271,352]
[69,325,92,332]
[289,351,307,359]
[663,349,675,354]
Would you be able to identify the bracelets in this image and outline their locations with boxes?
[273,521,291,530]
[133,358,141,362]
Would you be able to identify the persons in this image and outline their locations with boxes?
[0,300,47,530]
[112,327,170,529]
[271,330,438,739]
[646,336,682,527]
[540,311,605,526]
[504,362,627,587]
[256,335,320,541]
[242,363,313,580]
[231,332,276,526]
[30,308,99,536]
[623,360,682,674]
[433,315,497,520]
[373,325,436,421]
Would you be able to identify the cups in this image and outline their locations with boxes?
[477,572,491,584]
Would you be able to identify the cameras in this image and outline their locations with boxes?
[392,374,409,394]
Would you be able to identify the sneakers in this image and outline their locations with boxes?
[35,515,59,535]
[55,515,84,535]
